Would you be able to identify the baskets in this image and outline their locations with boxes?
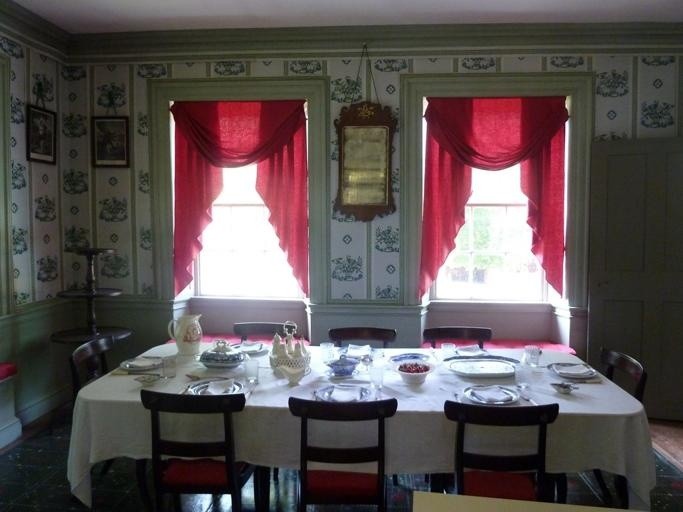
[269,354,311,378]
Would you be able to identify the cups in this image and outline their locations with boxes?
[163,343,540,390]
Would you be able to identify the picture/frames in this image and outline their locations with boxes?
[334,100,399,222]
[26,103,56,165]
[91,115,129,169]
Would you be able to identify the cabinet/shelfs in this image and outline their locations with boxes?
[50,247,132,421]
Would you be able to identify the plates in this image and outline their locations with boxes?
[120,339,597,405]
